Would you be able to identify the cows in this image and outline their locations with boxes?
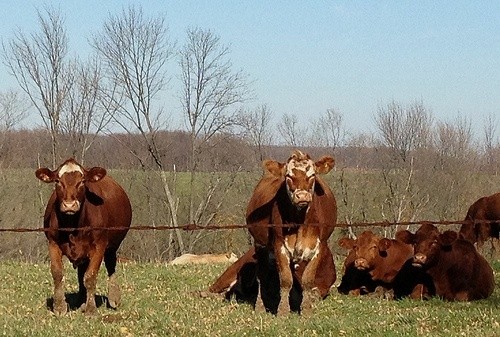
[336,192,500,301]
[34,161,132,318]
[210,148,337,318]
